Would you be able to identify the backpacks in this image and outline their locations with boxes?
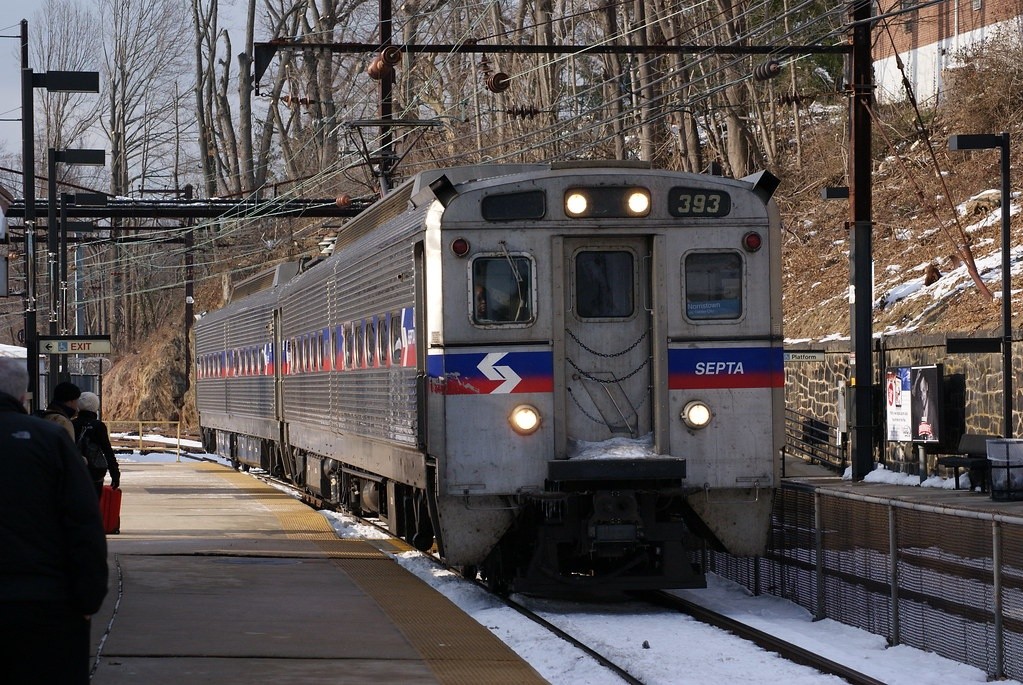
[30,406,70,425]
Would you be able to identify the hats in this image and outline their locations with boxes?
[77,391,100,413]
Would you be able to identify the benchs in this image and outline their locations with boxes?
[937,434,1003,491]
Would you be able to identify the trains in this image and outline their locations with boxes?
[193,160,783,592]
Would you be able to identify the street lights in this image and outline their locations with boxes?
[948,133,1013,439]
[22,69,108,416]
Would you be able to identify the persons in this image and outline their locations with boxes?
[70,392,120,503]
[0,356,109,684]
[40,382,88,465]
[476,285,495,321]
[912,377,938,440]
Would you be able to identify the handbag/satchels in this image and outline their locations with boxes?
[99,484,122,537]
[76,419,109,471]
